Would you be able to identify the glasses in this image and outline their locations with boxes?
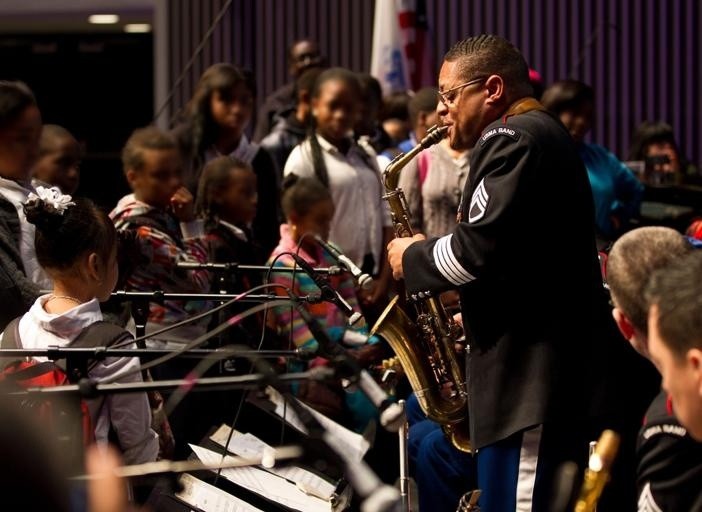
[437,75,485,104]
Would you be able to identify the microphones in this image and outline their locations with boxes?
[255,357,403,512]
[285,287,407,431]
[291,253,365,329]
[312,234,374,290]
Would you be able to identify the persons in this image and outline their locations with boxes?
[1,39,701,511]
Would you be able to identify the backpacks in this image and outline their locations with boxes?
[0,312,135,476]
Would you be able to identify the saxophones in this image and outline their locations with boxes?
[370,125,469,428]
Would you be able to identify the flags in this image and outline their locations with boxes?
[369,1,435,104]
